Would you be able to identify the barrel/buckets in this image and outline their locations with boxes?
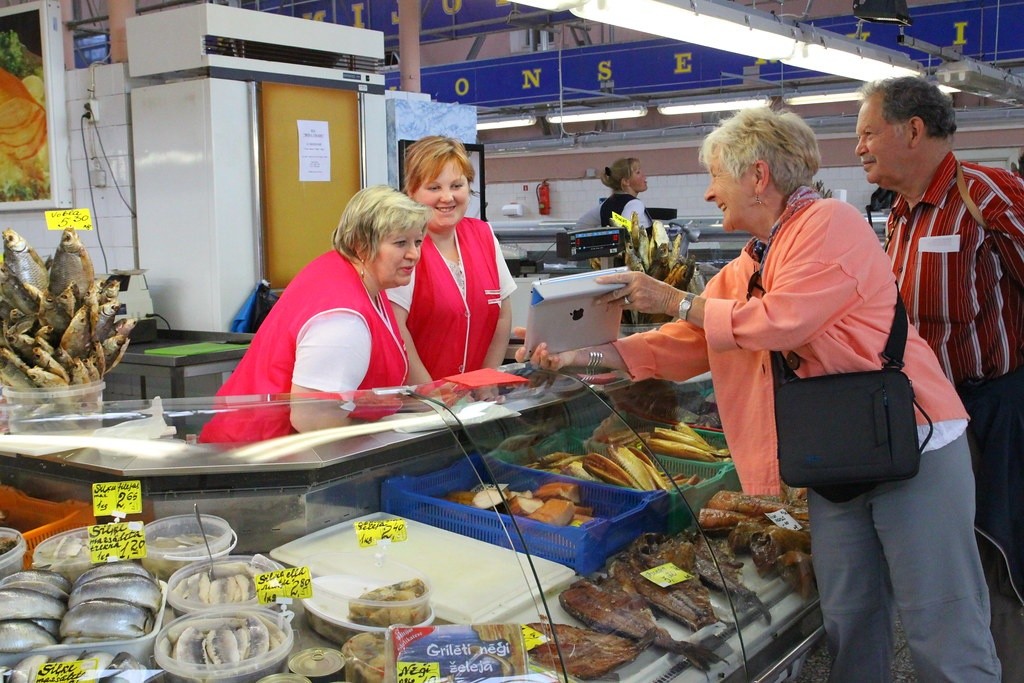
[2,381,105,444]
[2,512,295,681]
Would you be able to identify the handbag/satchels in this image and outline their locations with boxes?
[774,368,922,485]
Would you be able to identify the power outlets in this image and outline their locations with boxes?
[82,96,103,127]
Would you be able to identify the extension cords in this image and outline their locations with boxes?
[85,98,99,124]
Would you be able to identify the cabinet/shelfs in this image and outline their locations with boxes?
[0,320,834,682]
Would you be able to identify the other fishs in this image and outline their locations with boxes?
[587,211,709,337]
[0,560,286,683]
[0,227,139,417]
[524,476,817,681]
[523,419,733,491]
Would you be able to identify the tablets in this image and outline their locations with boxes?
[522,266,629,360]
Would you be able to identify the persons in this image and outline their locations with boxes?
[383,134,517,405]
[574,158,654,271]
[516,99,1003,683]
[853,76,1023,683]
[197,184,470,446]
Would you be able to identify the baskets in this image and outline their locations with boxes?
[490,433,727,538]
[621,397,725,431]
[569,411,744,494]
[383,452,668,578]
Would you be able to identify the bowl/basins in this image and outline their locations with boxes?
[296,551,437,645]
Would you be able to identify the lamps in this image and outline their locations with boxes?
[783,77,873,105]
[658,95,774,117]
[932,82,959,95]
[571,0,805,62]
[854,1,914,27]
[543,104,650,120]
[476,113,537,130]
[937,55,1024,106]
[781,24,926,87]
[508,0,591,20]
